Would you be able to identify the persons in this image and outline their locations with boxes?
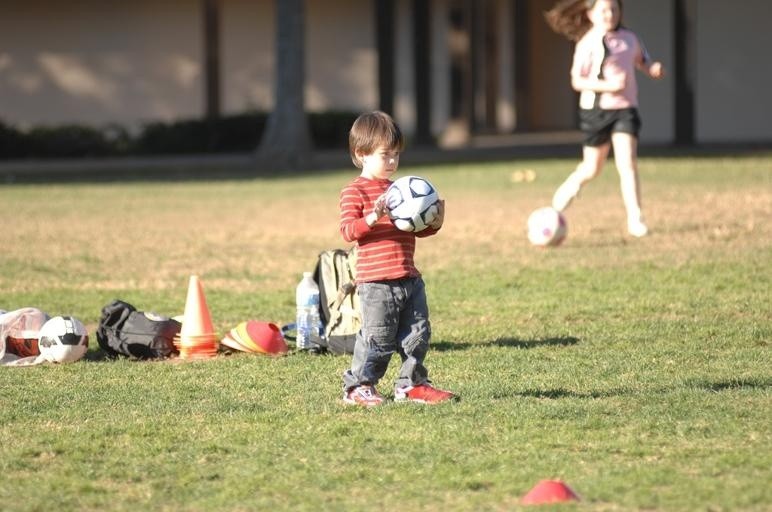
[545,0,665,240]
[337,109,459,409]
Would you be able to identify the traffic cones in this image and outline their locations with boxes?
[172,275,220,362]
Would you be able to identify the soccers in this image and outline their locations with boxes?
[385,176,439,232]
[38,316,89,364]
[527,207,567,246]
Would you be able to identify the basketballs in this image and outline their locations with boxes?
[7,311,52,357]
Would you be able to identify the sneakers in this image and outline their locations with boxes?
[343,386,385,409]
[629,217,646,241]
[395,384,456,405]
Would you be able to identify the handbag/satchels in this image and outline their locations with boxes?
[95,300,178,359]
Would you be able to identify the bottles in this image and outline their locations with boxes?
[296,271,320,352]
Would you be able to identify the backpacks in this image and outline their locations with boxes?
[312,248,362,354]
